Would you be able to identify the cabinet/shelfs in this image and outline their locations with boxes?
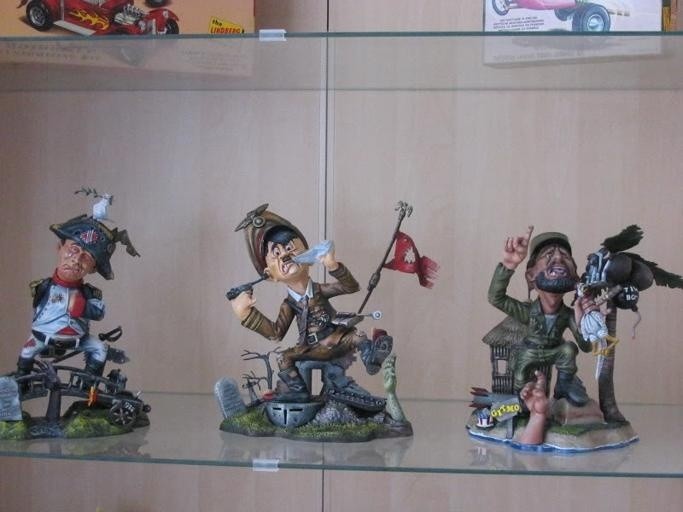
[0,27,680,479]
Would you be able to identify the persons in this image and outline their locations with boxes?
[461,224,681,453]
[0,186,149,442]
[211,199,441,442]
